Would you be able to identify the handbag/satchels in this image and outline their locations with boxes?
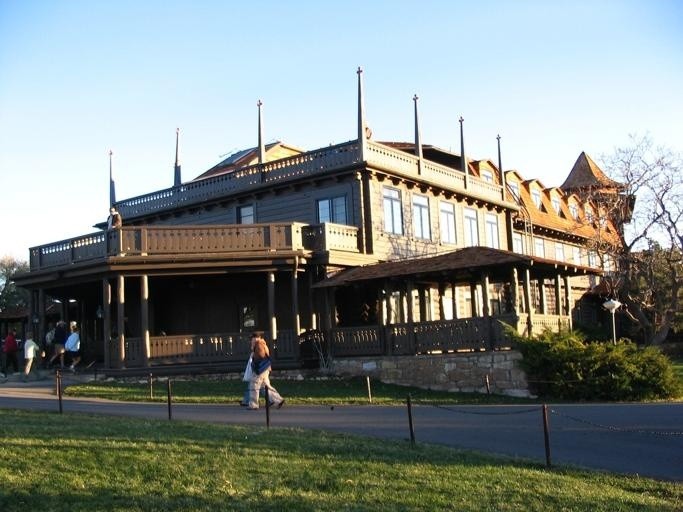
[251,357,272,374]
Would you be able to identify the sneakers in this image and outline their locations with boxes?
[240,402,259,410]
[269,400,285,409]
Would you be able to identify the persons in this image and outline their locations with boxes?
[2,329,21,378]
[24,332,41,377]
[65,326,82,373]
[247,333,285,411]
[50,320,66,372]
[106,207,122,230]
[240,337,275,407]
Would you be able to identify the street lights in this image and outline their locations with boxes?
[603,299,622,346]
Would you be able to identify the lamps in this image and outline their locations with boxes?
[31,312,39,328]
[95,304,104,319]
